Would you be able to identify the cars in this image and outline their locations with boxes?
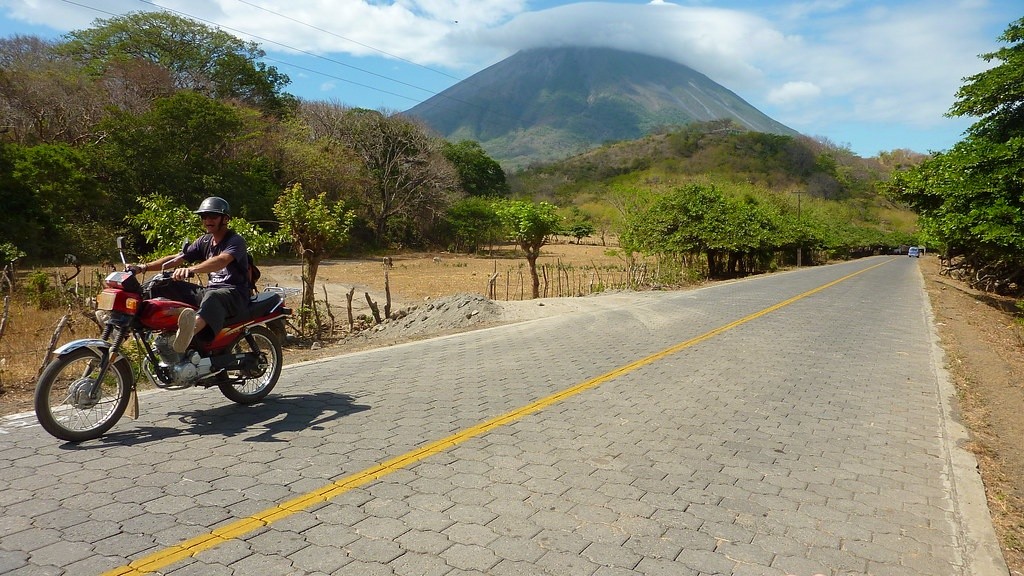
[908,247,920,258]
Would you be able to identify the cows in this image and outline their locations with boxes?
[382,257,394,270]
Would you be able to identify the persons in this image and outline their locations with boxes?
[95,196,250,354]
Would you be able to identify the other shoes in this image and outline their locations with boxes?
[172,307,197,353]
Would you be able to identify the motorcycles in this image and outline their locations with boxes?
[33,236,303,442]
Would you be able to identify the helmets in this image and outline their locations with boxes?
[193,196,232,221]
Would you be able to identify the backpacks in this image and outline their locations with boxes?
[201,229,260,296]
